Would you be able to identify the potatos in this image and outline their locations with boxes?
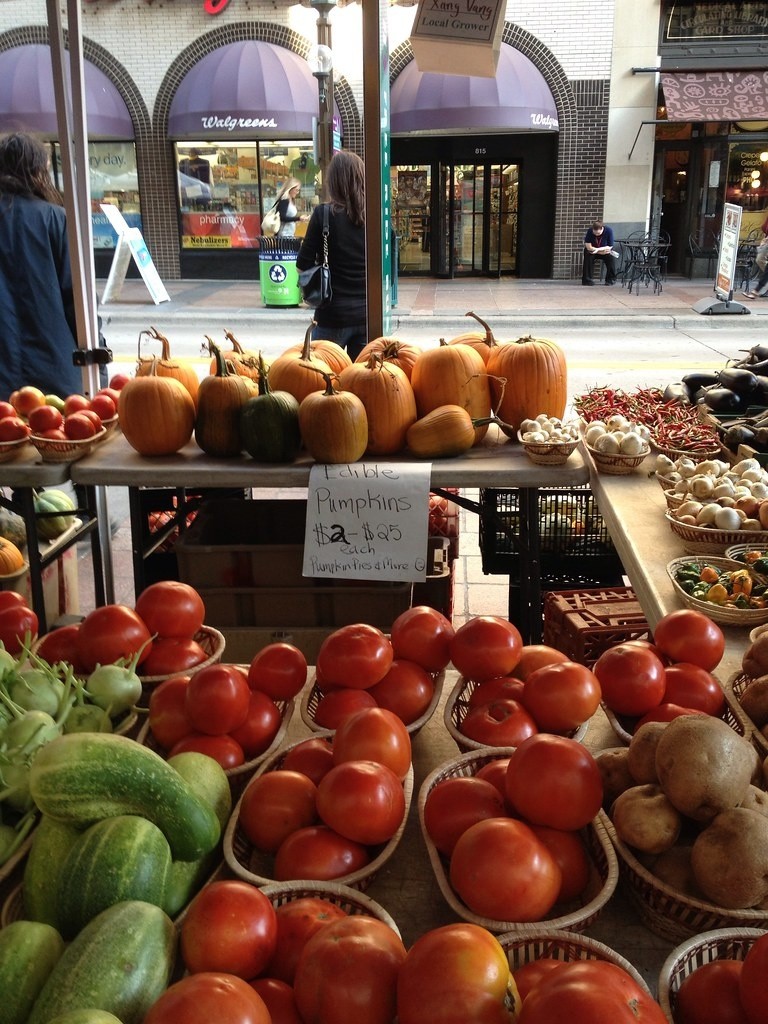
[593,631,768,914]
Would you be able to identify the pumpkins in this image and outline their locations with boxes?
[496,495,612,552]
[119,312,566,467]
[0,486,76,576]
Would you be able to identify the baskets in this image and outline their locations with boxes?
[0,373,768,1024]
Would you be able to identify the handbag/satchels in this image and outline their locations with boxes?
[261,202,281,234]
[297,204,333,305]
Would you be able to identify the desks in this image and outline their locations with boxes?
[72,404,590,645]
[569,419,768,682]
[739,238,755,276]
[625,243,671,294]
[741,243,760,288]
[0,425,122,635]
[613,239,654,283]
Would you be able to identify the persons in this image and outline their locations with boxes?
[296,152,367,363]
[275,177,310,236]
[741,217,768,299]
[581,221,616,286]
[179,155,211,211]
[0,133,119,541]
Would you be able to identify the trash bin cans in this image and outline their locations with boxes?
[257,237,302,308]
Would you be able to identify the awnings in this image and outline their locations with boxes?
[390,40,559,136]
[628,67,768,161]
[167,39,343,141]
[0,44,136,142]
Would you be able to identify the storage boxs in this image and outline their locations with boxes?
[0,483,656,668]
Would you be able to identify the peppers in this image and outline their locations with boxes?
[571,383,723,453]
[676,549,768,610]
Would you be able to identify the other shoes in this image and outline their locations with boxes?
[583,280,594,285]
[742,291,756,299]
[605,280,615,286]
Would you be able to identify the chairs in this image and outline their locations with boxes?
[584,228,766,296]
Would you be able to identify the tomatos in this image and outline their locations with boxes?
[149,493,201,552]
[0,375,130,444]
[144,881,767,1023]
[0,581,723,920]
[428,492,450,536]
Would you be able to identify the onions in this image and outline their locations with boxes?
[519,415,768,532]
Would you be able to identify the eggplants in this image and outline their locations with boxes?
[662,342,767,456]
